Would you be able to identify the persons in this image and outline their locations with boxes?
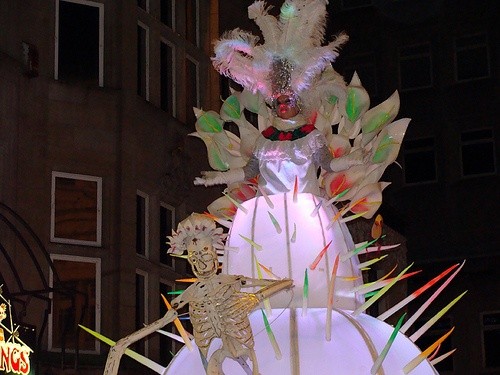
[192,78,374,198]
